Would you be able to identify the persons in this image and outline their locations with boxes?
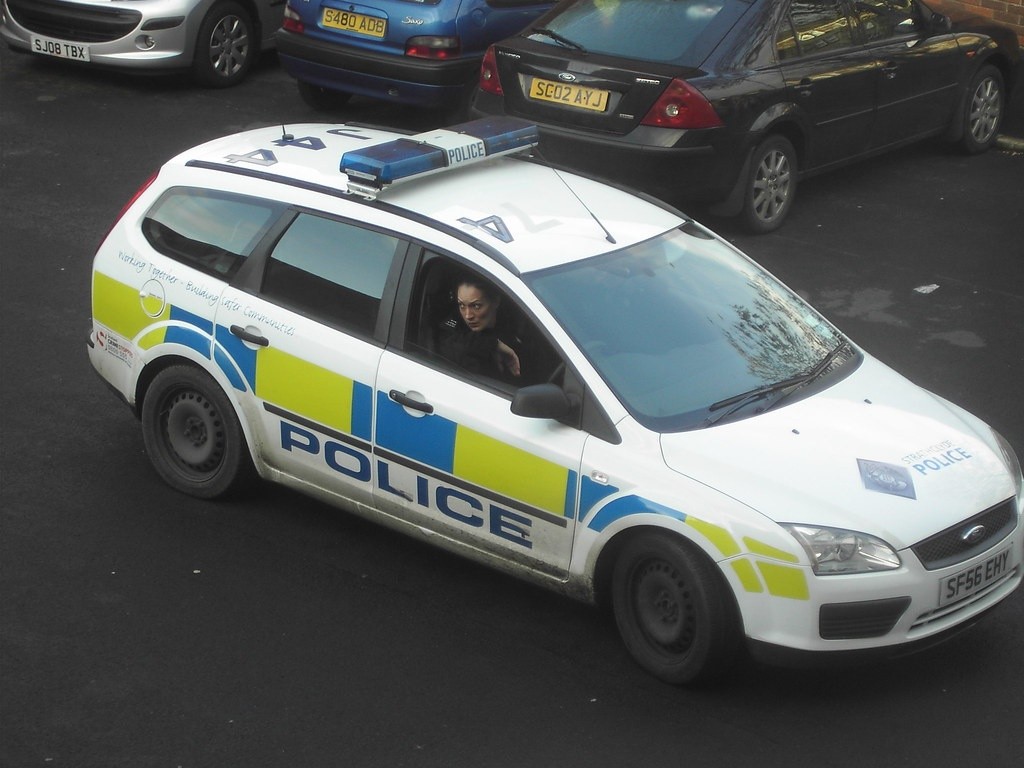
[440,275,531,382]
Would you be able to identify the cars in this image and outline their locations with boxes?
[469,0,1021,234]
[87,115,1024,688]
[0,0,286,89]
[274,0,563,112]
